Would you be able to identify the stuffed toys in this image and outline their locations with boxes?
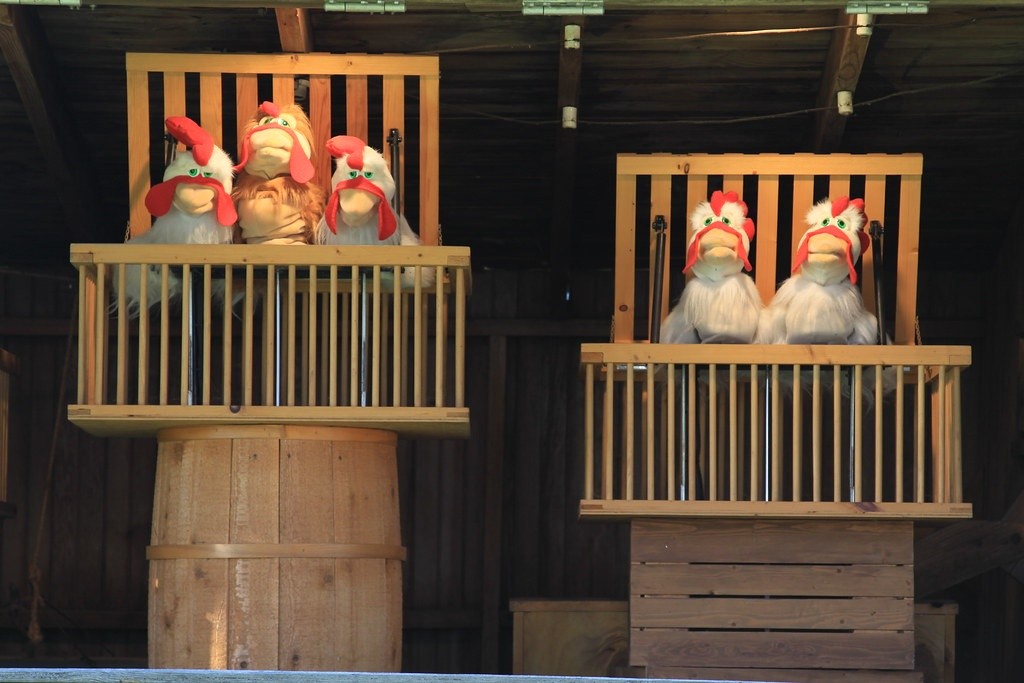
[113,115,245,319]
[316,135,435,292]
[777,195,898,407]
[659,189,789,376]
[233,101,324,245]
